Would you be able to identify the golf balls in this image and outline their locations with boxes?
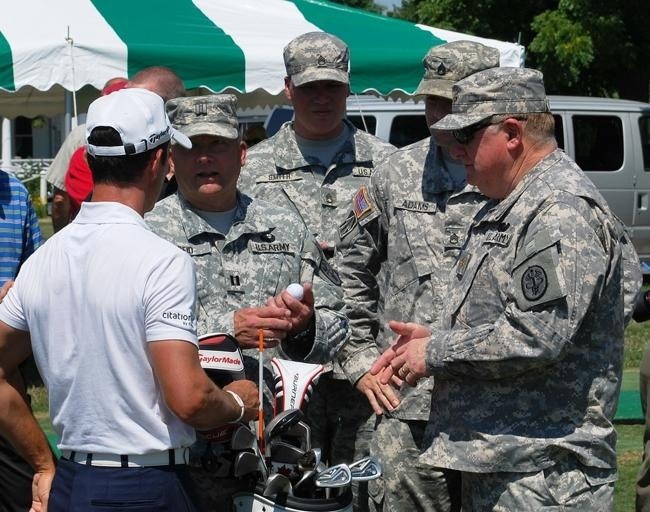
[287,282,303,300]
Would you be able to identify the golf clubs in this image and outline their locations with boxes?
[231,408,382,501]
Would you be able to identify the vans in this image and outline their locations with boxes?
[265,95,650,261]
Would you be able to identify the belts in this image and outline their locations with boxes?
[62,448,192,467]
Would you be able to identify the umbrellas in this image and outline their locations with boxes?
[0,0,527,101]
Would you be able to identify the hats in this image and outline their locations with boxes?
[83,89,193,155]
[63,146,93,203]
[283,30,352,88]
[166,93,244,147]
[431,66,549,132]
[413,40,500,102]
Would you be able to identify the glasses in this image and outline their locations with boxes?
[449,117,527,144]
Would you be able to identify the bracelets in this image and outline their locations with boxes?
[225,390,245,424]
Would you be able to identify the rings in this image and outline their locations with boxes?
[401,364,409,374]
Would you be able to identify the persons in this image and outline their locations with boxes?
[0,167,46,512]
[369,67,621,512]
[44,67,187,234]
[235,30,401,512]
[632,282,650,511]
[1,85,260,512]
[333,40,644,511]
[142,94,350,510]
